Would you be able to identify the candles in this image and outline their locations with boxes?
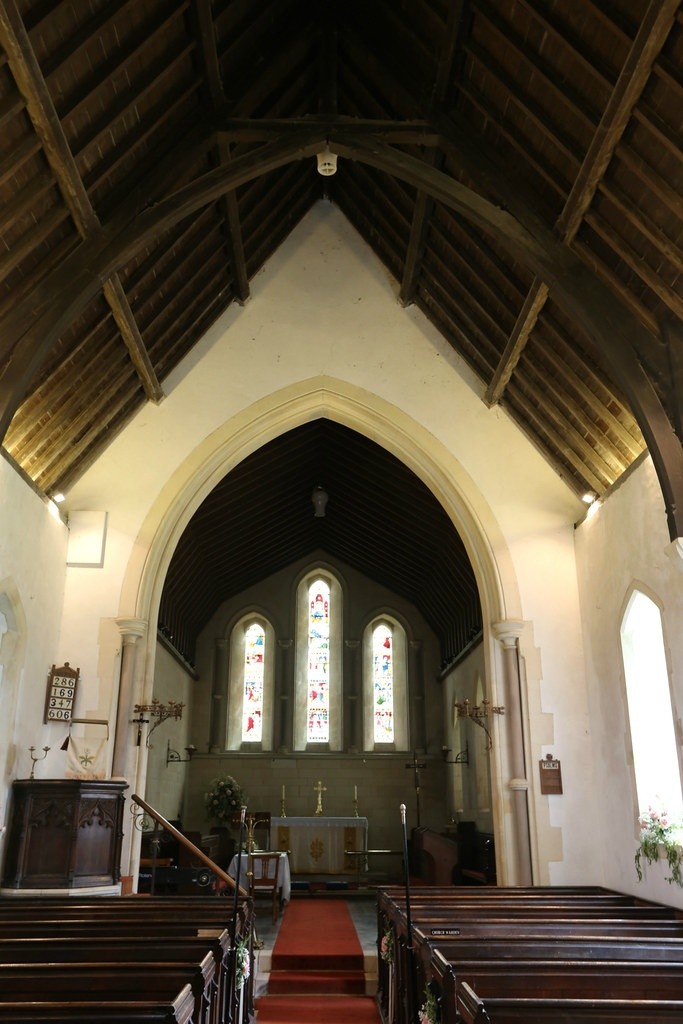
[355,784,357,800]
[282,785,285,800]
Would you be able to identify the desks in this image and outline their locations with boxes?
[227,850,291,912]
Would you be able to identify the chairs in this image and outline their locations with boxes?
[251,854,281,925]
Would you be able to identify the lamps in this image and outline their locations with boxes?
[317,135,338,175]
[166,740,197,767]
[441,740,468,765]
[312,486,328,516]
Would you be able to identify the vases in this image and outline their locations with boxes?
[210,827,231,865]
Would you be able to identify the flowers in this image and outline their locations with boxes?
[635,806,683,884]
[236,934,250,990]
[380,928,393,964]
[204,775,245,825]
[417,985,438,1024]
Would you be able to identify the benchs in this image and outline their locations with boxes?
[375,886,683,1024]
[0,892,256,1024]
